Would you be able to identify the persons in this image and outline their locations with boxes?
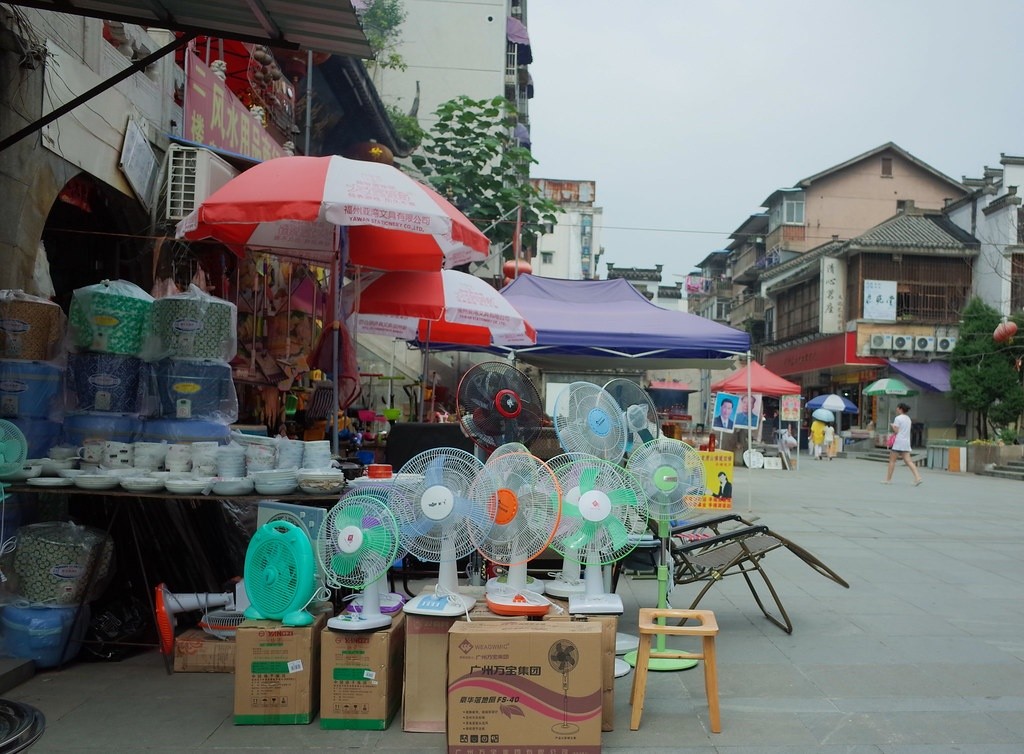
[808,421,834,460]
[735,395,757,427]
[714,398,733,430]
[770,406,791,459]
[879,403,923,487]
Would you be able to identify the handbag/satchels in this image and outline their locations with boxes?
[808,437,813,455]
[887,434,896,447]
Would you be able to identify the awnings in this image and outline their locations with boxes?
[515,123,530,147]
[507,16,532,66]
[884,358,950,393]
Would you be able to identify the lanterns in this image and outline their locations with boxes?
[994,321,1017,344]
[353,139,393,165]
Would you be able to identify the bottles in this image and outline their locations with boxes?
[655,402,687,416]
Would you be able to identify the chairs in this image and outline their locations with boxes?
[629,498,849,634]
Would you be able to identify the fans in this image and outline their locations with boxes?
[244,360,707,677]
[155,585,246,655]
[0,420,28,501]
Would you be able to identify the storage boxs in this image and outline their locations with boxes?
[174,586,619,754]
[623,541,661,570]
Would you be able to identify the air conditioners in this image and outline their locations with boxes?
[870,333,956,353]
[166,146,242,220]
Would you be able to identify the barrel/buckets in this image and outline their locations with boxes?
[1,290,236,458]
[0,521,114,668]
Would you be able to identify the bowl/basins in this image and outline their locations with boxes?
[10,440,393,478]
[27,468,427,495]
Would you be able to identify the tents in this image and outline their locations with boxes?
[413,272,753,513]
[711,361,801,470]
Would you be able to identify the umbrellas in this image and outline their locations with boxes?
[862,378,919,450]
[174,154,492,459]
[805,394,858,414]
[345,269,538,423]
[812,408,834,422]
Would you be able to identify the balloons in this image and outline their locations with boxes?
[503,259,532,285]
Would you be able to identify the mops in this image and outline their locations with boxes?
[221,254,334,426]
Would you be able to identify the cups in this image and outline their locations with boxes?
[700,444,707,451]
[709,434,715,451]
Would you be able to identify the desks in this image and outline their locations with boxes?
[682,446,735,510]
[9,482,415,677]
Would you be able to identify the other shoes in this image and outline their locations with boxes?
[829,456,832,460]
[881,479,892,484]
[815,456,819,460]
[914,478,922,487]
[819,455,823,460]
